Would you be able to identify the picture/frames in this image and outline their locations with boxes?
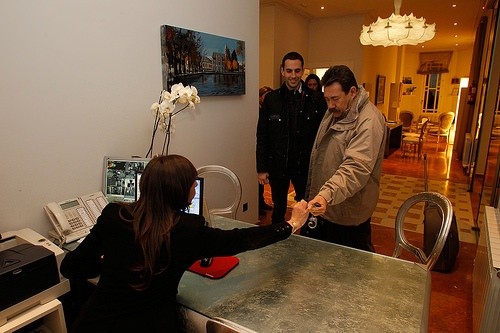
[101,156,153,203]
[375,75,386,107]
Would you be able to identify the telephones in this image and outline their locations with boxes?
[43,197,96,245]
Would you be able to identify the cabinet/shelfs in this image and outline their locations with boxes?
[384,124,402,159]
[402,84,416,96]
[0,299,67,333]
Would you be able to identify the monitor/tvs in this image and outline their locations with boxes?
[103,156,153,201]
[181,178,205,215]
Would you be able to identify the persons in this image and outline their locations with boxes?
[253,51,327,224]
[58,154,309,333]
[304,65,387,253]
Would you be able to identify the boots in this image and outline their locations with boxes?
[258,184,273,216]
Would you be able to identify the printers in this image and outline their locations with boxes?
[0,227,71,327]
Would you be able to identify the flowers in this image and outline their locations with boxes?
[144,82,201,159]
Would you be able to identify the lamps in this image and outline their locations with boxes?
[359,0,436,48]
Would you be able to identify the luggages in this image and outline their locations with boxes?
[424,155,460,273]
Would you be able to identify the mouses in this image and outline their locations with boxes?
[200,256,213,267]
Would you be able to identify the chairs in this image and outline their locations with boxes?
[392,192,452,272]
[196,165,242,220]
[50,214,431,333]
[399,110,455,160]
